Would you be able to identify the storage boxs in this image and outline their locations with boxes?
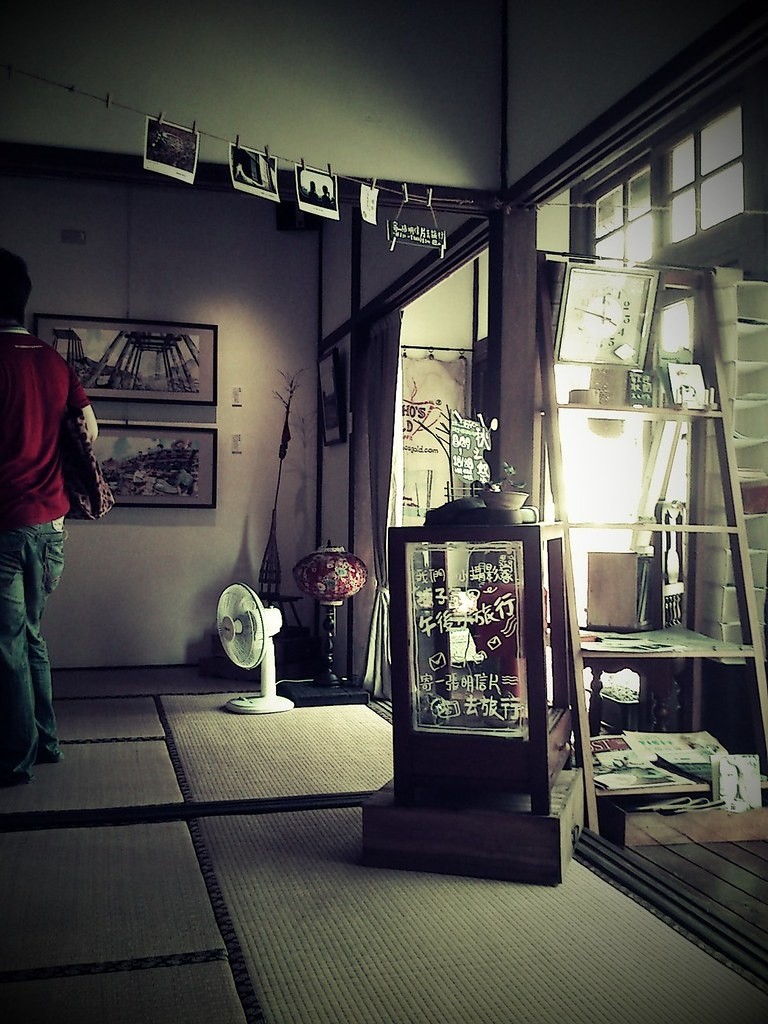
[599,804,768,848]
[586,552,655,633]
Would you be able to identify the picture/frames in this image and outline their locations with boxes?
[93,423,218,508]
[317,347,346,447]
[31,313,218,406]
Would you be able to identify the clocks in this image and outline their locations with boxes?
[555,263,660,367]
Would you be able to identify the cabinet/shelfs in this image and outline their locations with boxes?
[386,524,572,815]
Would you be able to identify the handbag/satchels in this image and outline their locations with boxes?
[58,360,116,521]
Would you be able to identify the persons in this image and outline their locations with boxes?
[720,757,753,811]
[99,441,199,497]
[308,181,318,203]
[0,247,97,785]
[322,186,331,207]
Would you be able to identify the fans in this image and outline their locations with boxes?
[215,582,295,715]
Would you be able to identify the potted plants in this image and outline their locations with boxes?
[478,463,531,511]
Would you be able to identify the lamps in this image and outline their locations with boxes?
[293,539,368,690]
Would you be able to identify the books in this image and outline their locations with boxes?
[572,731,768,811]
[637,559,650,622]
